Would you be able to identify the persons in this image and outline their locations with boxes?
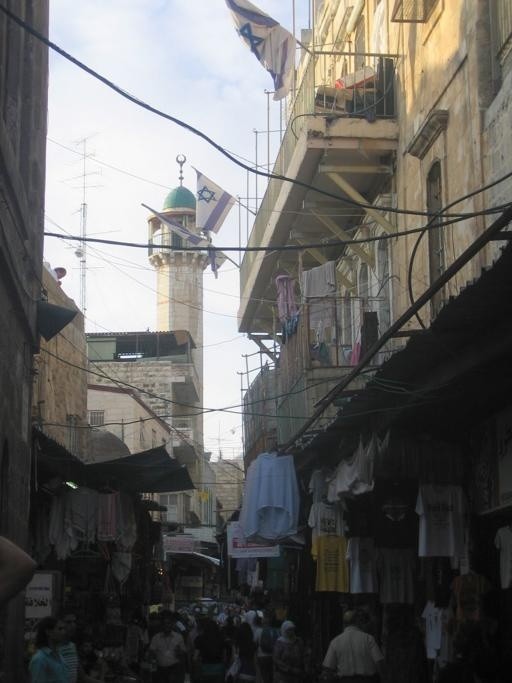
[0,535,40,609]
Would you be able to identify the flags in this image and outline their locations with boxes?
[154,212,228,279]
[225,0,298,101]
[195,171,237,233]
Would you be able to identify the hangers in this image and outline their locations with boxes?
[268,444,280,456]
